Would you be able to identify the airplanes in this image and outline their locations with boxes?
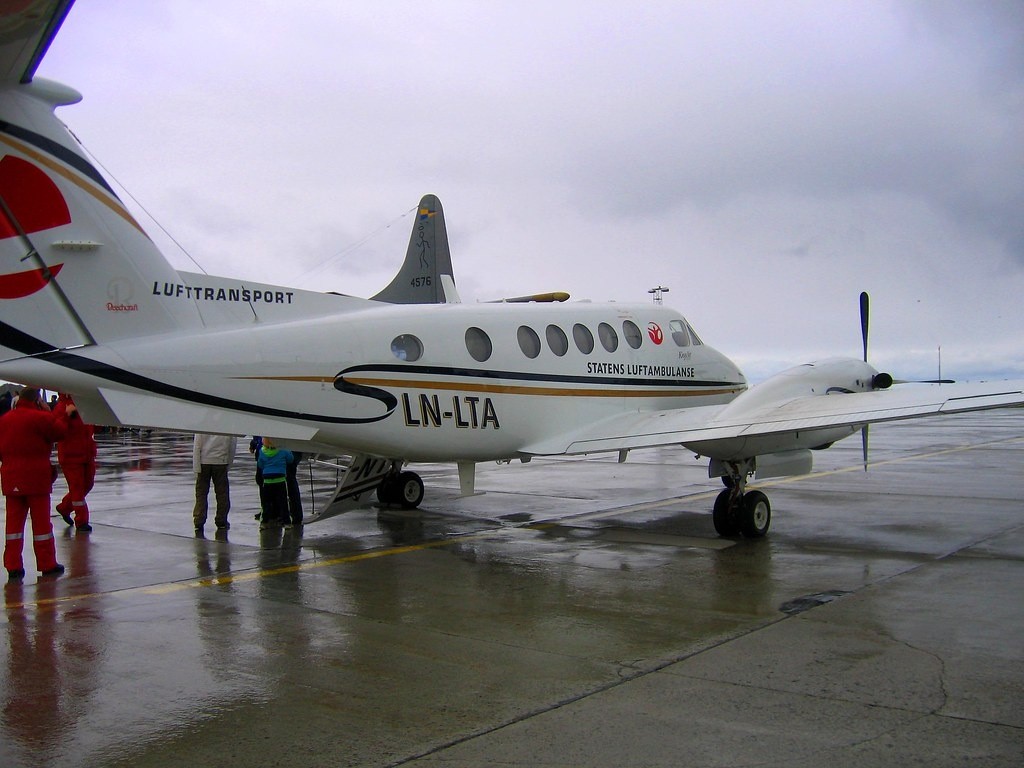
[0,0,1024,543]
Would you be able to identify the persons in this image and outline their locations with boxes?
[192,432,302,536]
[0,385,99,580]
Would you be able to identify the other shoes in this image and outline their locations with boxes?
[55,505,74,526]
[8,567,25,579]
[194,524,203,531]
[42,563,66,575]
[258,523,271,529]
[254,512,262,518]
[218,523,231,529]
[284,523,294,529]
[76,523,93,531]
